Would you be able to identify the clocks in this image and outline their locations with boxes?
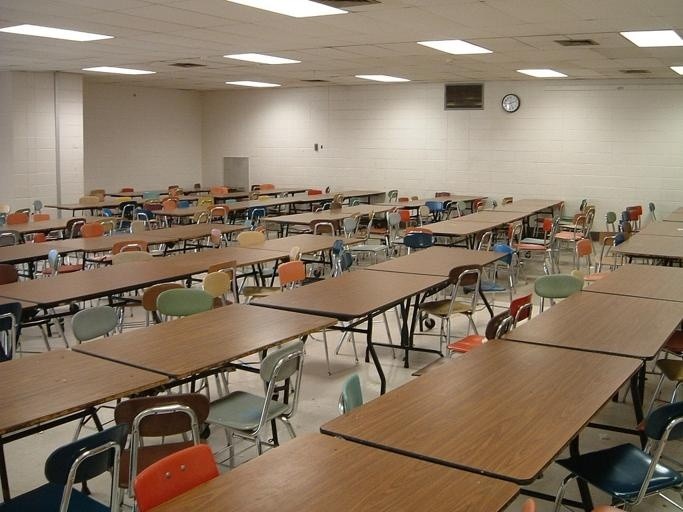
[501,93,521,114]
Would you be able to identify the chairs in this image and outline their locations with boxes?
[0,175,682,512]
